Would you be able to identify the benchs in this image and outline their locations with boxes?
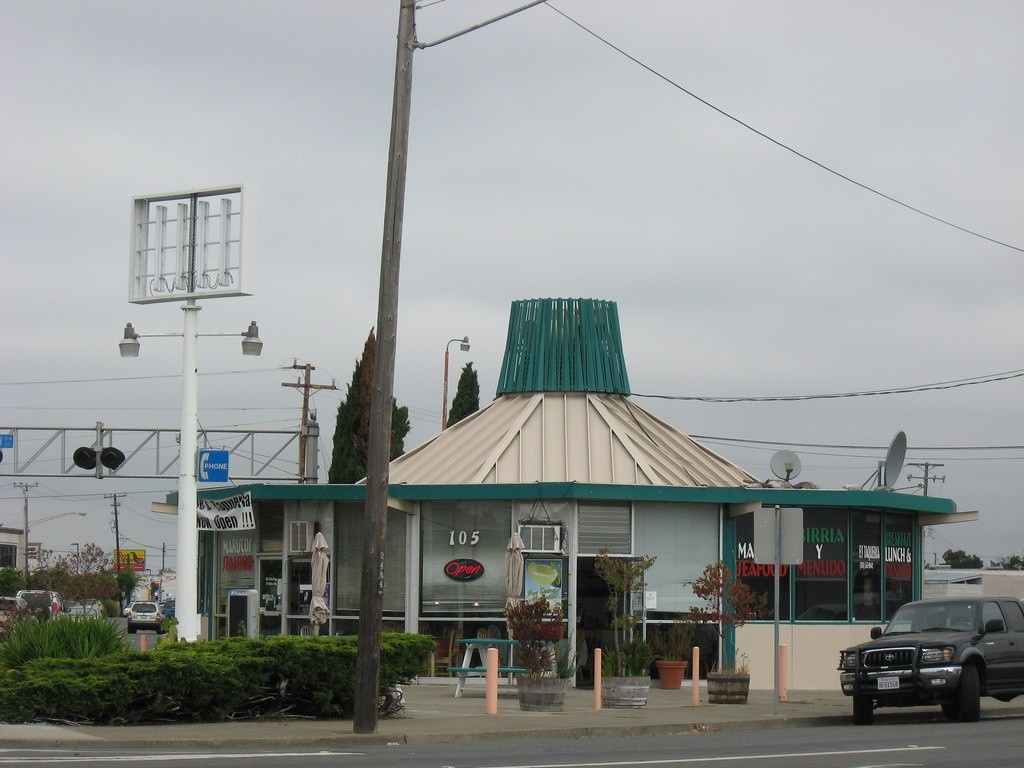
[448,666,545,698]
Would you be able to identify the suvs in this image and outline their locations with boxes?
[837,596,1023,726]
[15,589,64,623]
[127,601,167,633]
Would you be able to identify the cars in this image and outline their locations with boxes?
[123,602,135,618]
[795,601,906,621]
[160,600,175,618]
[0,596,39,642]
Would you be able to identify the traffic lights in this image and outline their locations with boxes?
[100,446,127,470]
[74,446,99,470]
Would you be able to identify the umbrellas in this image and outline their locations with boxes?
[307,532,330,635]
[503,533,526,686]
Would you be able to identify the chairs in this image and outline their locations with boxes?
[300,626,315,636]
[381,622,507,678]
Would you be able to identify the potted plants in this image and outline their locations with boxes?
[687,560,775,705]
[505,593,584,712]
[594,543,657,709]
[649,611,697,689]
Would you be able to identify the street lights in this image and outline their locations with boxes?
[25,512,86,589]
[442,336,469,431]
[120,297,265,642]
[71,542,79,576]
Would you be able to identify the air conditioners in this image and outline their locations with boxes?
[290,520,315,552]
[518,525,563,553]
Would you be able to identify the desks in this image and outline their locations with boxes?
[454,639,568,698]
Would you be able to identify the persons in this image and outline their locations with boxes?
[322,592,328,606]
[301,591,310,605]
